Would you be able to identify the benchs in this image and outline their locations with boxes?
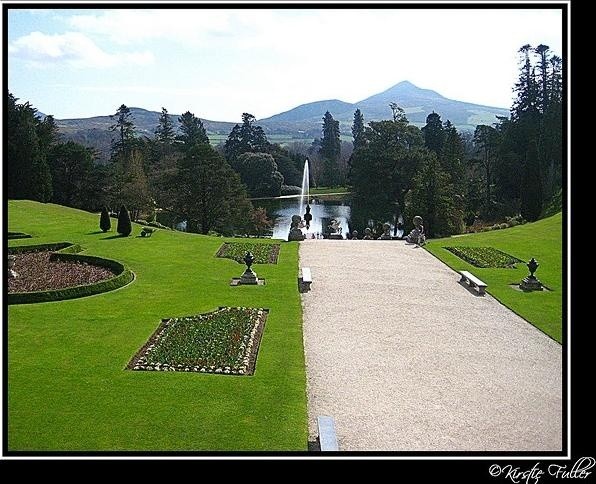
[317,416,338,451]
[301,266,312,291]
[459,270,488,296]
